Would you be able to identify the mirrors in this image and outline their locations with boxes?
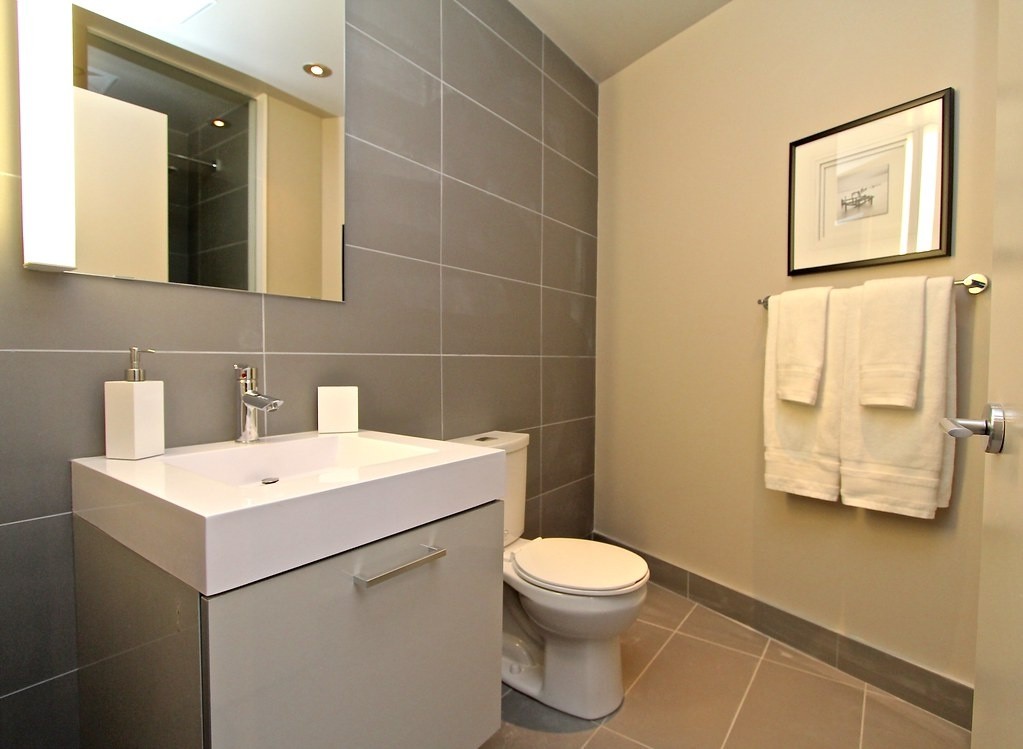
[14,0,347,307]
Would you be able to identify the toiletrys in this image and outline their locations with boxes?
[99,346,174,464]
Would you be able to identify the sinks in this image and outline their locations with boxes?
[70,425,504,598]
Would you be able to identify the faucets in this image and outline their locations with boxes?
[230,360,289,444]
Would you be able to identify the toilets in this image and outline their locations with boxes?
[444,427,652,722]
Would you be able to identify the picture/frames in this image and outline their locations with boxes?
[785,87,956,277]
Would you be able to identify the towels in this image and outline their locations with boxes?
[856,275,929,412]
[838,275,960,521]
[760,287,851,508]
[772,284,834,407]
